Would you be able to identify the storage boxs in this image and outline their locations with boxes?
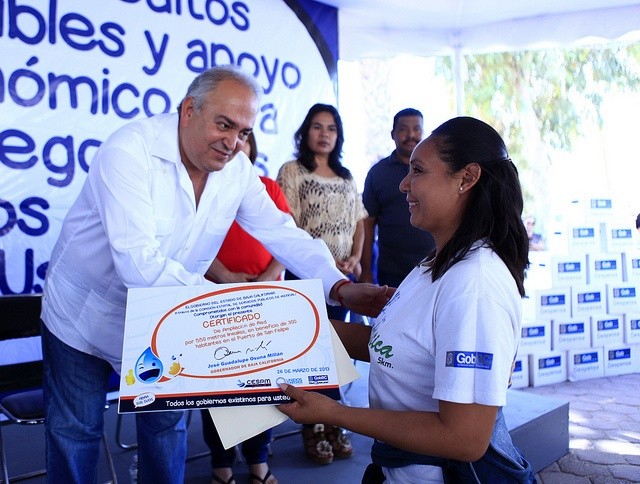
[508,194,639,390]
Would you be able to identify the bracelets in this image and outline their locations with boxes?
[336,280,352,305]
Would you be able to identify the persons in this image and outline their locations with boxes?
[276,116,537,484]
[39,66,398,484]
[200,127,294,484]
[360,108,436,289]
[277,103,369,464]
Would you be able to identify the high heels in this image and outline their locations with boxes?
[301,422,333,464]
[325,423,352,459]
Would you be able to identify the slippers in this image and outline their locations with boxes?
[249,470,278,484]
[211,471,236,484]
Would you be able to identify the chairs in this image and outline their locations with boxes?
[0,294,118,484]
